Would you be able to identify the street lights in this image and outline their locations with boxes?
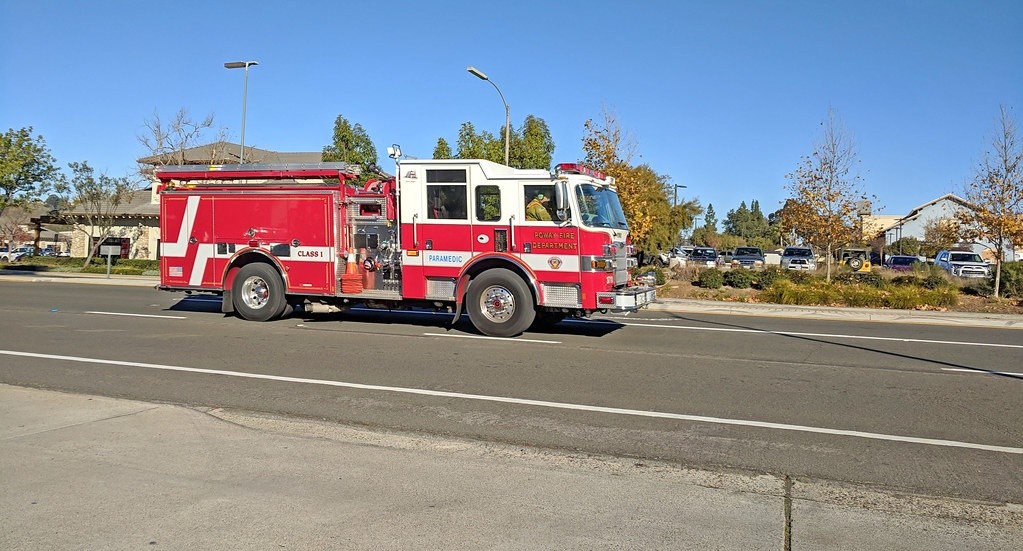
[672,183,688,258]
[466,66,512,167]
[224,61,260,165]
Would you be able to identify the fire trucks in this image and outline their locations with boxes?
[154,143,658,339]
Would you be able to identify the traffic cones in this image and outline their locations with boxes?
[341,246,364,294]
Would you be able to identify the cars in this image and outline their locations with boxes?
[0,246,70,263]
[883,256,923,272]
[649,245,735,268]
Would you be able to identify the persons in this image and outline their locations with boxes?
[525,190,559,221]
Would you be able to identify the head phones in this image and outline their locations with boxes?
[538,188,545,200]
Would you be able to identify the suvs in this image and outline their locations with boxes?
[779,247,818,273]
[934,248,992,279]
[729,246,767,268]
[838,249,871,276]
